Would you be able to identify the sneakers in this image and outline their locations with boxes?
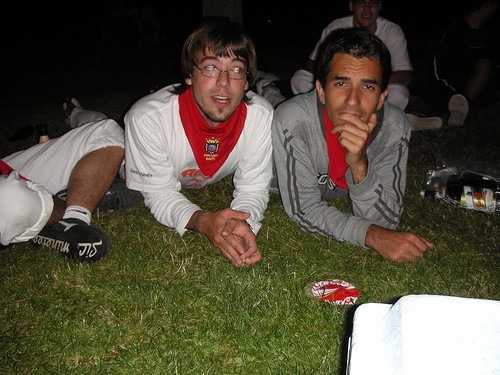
[31,218,108,262]
[55,177,144,210]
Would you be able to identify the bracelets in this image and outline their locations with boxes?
[194,209,206,233]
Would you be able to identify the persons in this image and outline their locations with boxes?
[1,111,125,261]
[54,19,276,269]
[246,27,438,263]
[291,0,416,132]
[410,0,500,131]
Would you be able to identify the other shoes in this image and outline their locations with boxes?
[448,94,470,128]
[404,110,444,131]
[255,77,275,96]
[62,97,81,125]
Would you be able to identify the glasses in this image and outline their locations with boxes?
[354,0,378,8]
[194,62,250,80]
